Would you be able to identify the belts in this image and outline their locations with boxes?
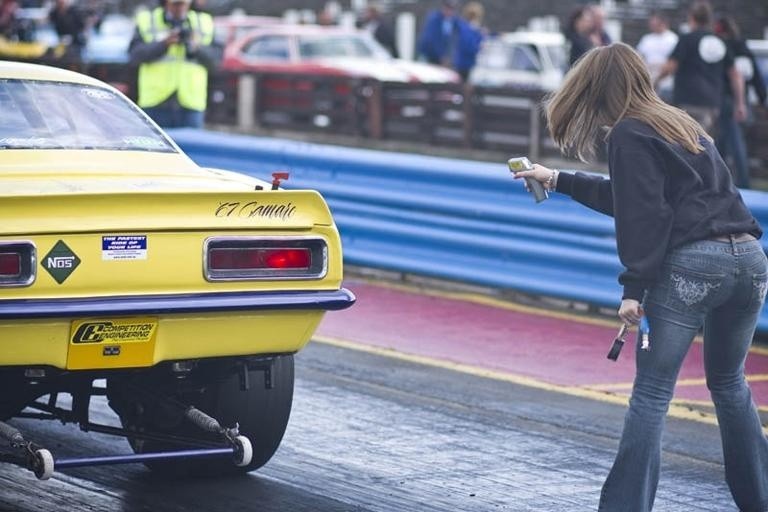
[710,230,758,244]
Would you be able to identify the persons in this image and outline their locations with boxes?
[636,8,680,104]
[587,1,612,45]
[413,1,483,83]
[713,17,758,153]
[314,1,399,58]
[2,1,148,74]
[126,2,225,131]
[514,43,768,512]
[461,1,500,39]
[653,2,746,160]
[564,7,604,66]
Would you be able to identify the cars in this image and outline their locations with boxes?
[219,24,468,136]
[0,56,361,487]
[214,14,289,48]
[465,29,573,122]
